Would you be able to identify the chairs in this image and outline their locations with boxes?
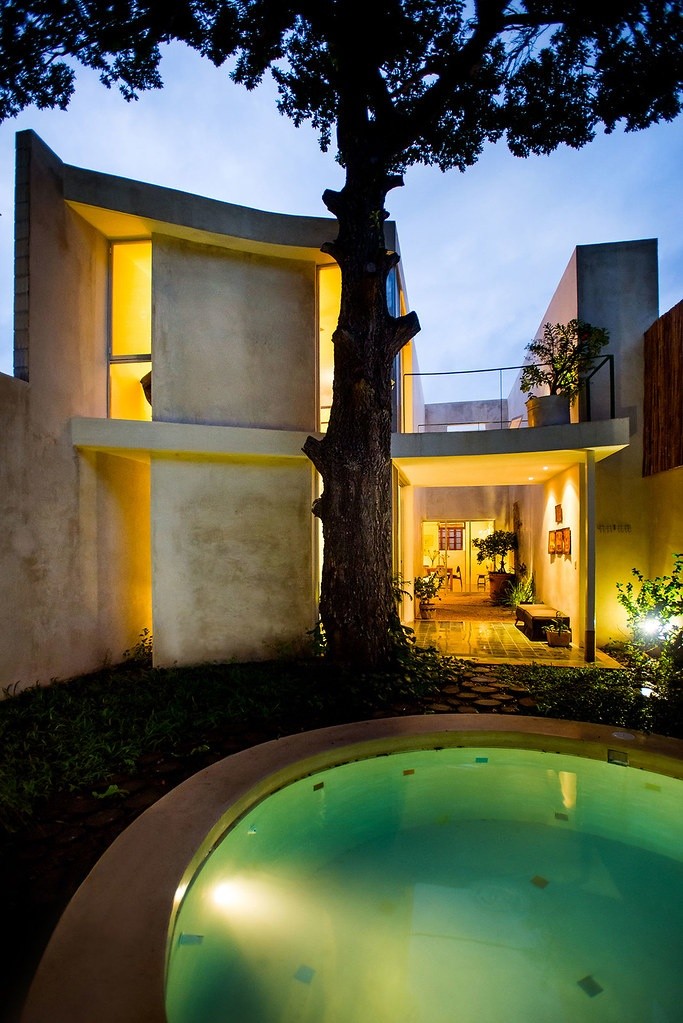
[447,566,462,587]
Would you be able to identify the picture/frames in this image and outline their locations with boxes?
[555,503,563,522]
[548,527,572,554]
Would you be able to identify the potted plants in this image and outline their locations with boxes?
[516,320,612,429]
[503,574,535,619]
[541,610,571,647]
[471,530,519,599]
[415,569,445,619]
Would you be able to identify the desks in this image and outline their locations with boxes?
[425,567,453,591]
[513,603,570,642]
